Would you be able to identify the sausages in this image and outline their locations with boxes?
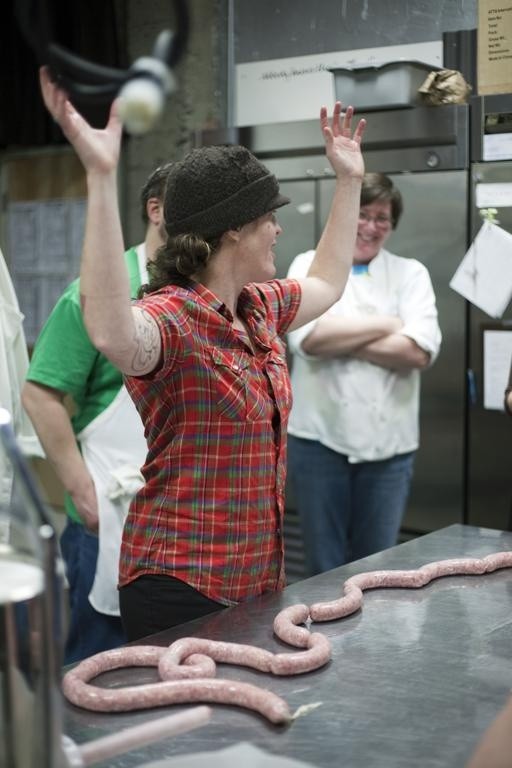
[61,551,512,723]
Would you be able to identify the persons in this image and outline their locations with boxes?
[39,62,368,648]
[19,160,177,667]
[283,172,445,582]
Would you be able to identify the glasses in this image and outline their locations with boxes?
[358,210,397,230]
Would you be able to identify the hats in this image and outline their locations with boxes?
[160,143,291,245]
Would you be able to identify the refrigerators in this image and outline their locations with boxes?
[469,93,512,529]
[191,99,469,598]
